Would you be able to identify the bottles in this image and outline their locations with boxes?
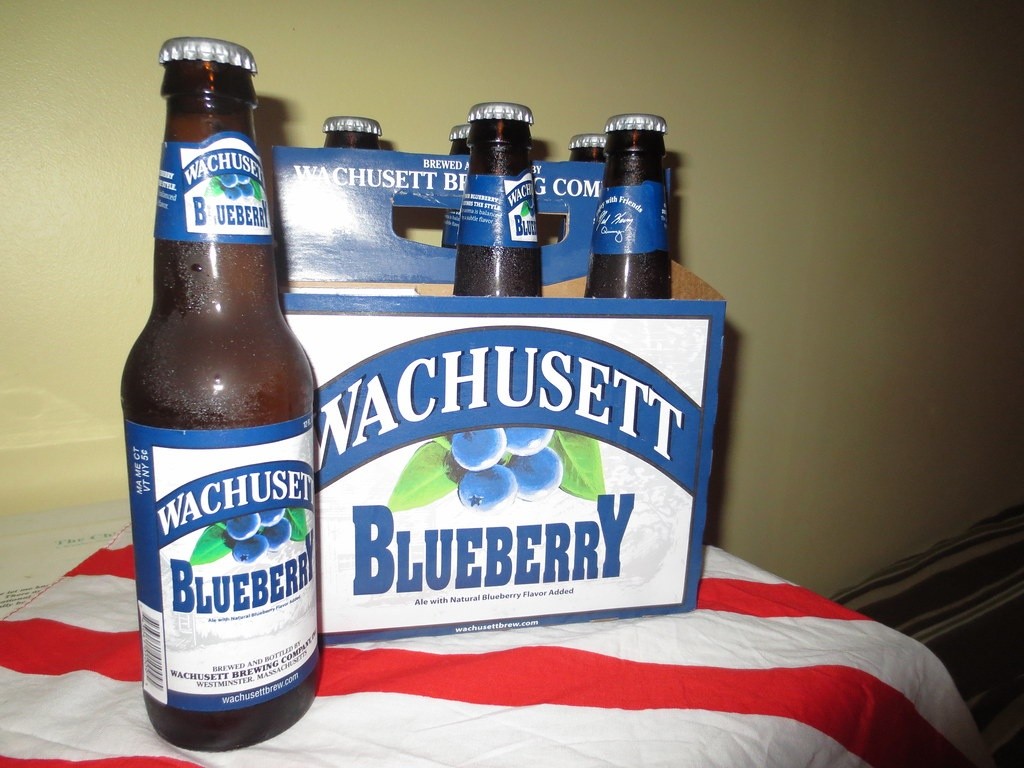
[442,123,470,249]
[558,134,606,243]
[322,116,382,151]
[584,113,671,298]
[453,102,543,296]
[123,36,318,751]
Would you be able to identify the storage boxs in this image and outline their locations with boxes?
[263,145,727,644]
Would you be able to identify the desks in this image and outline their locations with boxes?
[0,520,985,768]
